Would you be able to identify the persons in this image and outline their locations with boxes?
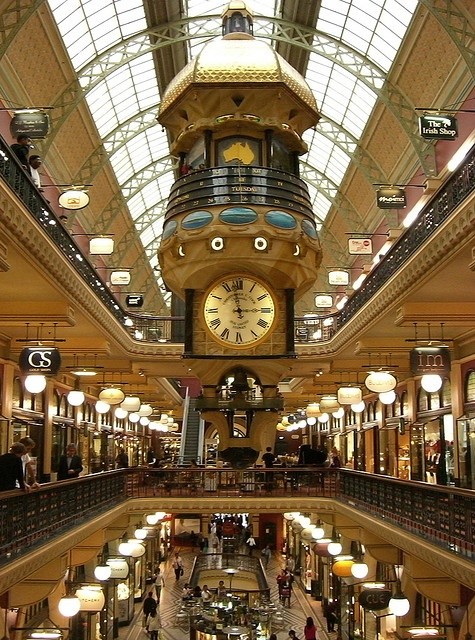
[143,591,157,633]
[201,585,212,606]
[276,569,285,600]
[115,448,128,468]
[158,456,172,479]
[187,163,205,174]
[172,553,182,581]
[266,634,277,640]
[0,442,29,494]
[57,215,68,226]
[153,568,165,604]
[211,533,219,554]
[189,459,198,477]
[148,448,159,467]
[10,133,32,195]
[194,586,202,597]
[305,551,311,571]
[28,154,50,211]
[217,581,227,596]
[287,555,296,574]
[261,545,272,570]
[15,436,41,492]
[57,443,83,480]
[262,447,275,485]
[210,513,249,533]
[326,600,338,633]
[287,630,300,640]
[183,583,191,600]
[146,605,161,640]
[281,567,294,609]
[246,534,257,556]
[304,617,318,640]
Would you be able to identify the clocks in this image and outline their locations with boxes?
[199,273,277,352]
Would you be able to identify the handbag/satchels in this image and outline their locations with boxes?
[142,615,147,627]
[277,580,281,584]
[261,553,265,556]
[181,569,183,576]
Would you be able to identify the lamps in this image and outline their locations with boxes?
[327,530,342,556]
[66,376,86,406]
[286,512,312,530]
[311,519,324,540]
[388,577,411,617]
[57,570,81,618]
[119,536,135,557]
[147,509,165,524]
[134,521,147,540]
[352,546,369,579]
[94,551,111,581]
